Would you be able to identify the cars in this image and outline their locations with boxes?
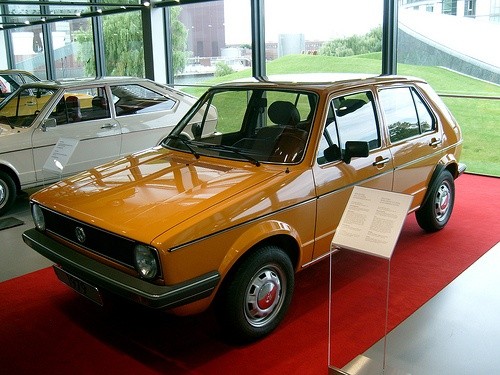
[0,75,219,217]
[21,72,467,338]
[0,70,96,117]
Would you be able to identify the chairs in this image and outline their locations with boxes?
[65,96,82,122]
[243,101,309,164]
[84,97,109,120]
